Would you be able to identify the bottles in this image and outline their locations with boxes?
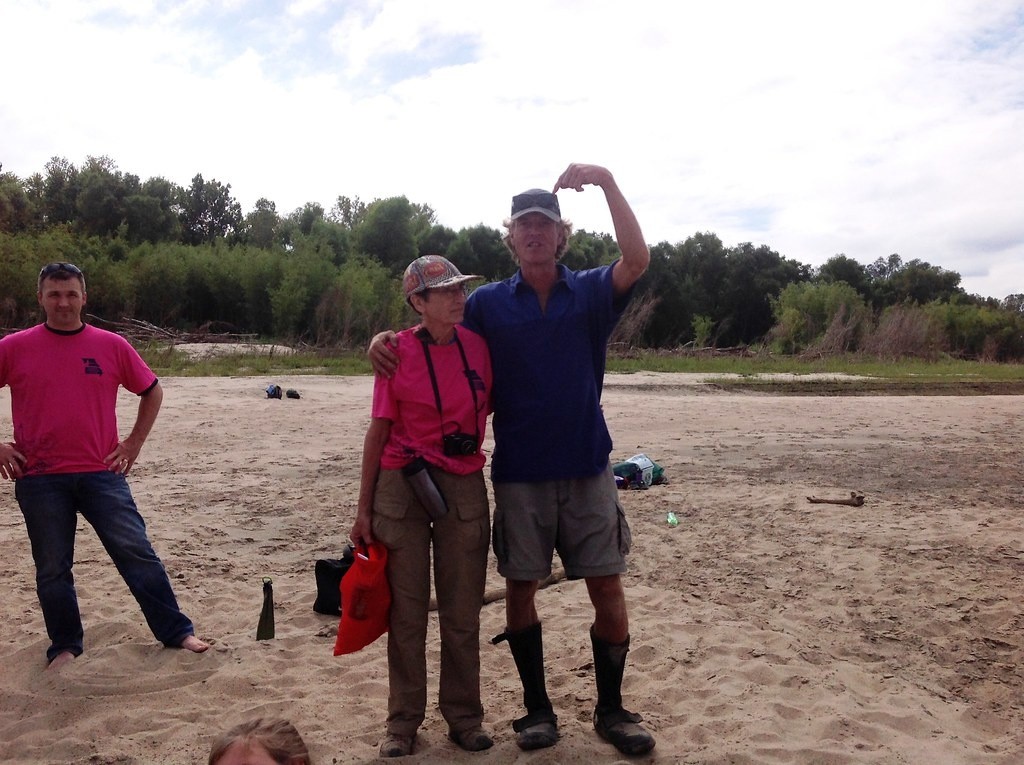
[667,512,677,525]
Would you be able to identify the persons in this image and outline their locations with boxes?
[368,161,662,754]
[0,264,210,668]
[351,255,495,755]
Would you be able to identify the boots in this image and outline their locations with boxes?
[586,623,657,757]
[491,620,559,751]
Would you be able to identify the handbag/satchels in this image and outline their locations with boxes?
[309,545,391,657]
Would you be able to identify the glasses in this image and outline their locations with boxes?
[39,262,84,279]
[424,286,469,299]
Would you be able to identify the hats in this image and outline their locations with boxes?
[401,254,485,299]
[511,188,562,224]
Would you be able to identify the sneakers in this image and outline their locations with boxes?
[447,724,497,752]
[378,730,418,758]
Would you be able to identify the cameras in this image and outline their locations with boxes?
[442,434,477,457]
